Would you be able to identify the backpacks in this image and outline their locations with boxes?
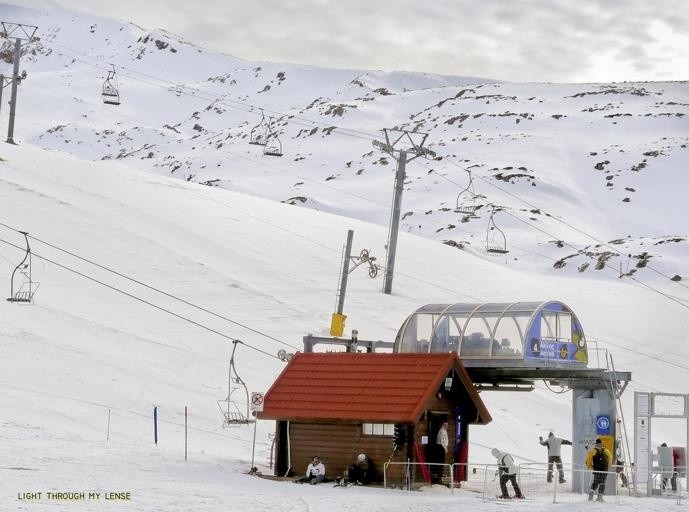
[592,448,608,471]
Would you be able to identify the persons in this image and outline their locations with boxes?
[293,456,326,485]
[430,421,450,485]
[491,446,523,499]
[661,442,682,494]
[584,439,613,502]
[539,433,572,483]
[616,440,628,487]
[346,453,376,487]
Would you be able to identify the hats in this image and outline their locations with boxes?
[595,439,601,444]
[358,453,366,462]
